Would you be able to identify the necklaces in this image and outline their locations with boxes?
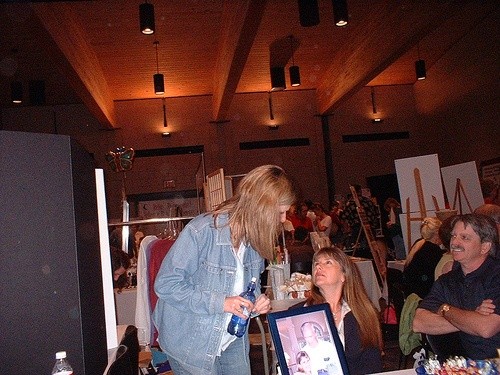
[332,310,340,320]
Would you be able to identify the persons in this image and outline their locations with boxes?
[111,246,130,280]
[403,179,500,359]
[289,248,384,375]
[296,322,343,375]
[279,194,351,274]
[151,166,295,375]
[340,185,379,259]
[384,198,405,260]
[110,218,136,258]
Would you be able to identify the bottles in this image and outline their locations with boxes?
[227,278,256,338]
[50,350,74,375]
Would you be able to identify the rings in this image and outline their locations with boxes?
[242,301,244,305]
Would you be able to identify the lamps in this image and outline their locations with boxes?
[415,41,426,81]
[151,42,165,94]
[332,0,348,27]
[287,35,301,87]
[139,0,156,36]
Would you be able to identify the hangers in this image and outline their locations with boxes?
[157,219,179,241]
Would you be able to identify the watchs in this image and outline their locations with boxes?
[443,305,450,316]
